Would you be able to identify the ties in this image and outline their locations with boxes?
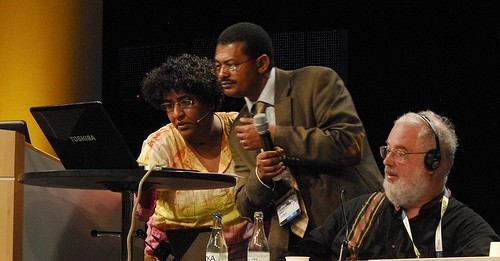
[255,102,309,238]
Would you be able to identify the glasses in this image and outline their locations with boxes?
[160,98,203,112]
[212,55,261,74]
[379,145,429,164]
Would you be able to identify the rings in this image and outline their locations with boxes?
[242,140,246,145]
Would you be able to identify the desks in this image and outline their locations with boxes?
[18,170,237,261]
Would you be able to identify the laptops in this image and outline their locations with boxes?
[29,101,200,172]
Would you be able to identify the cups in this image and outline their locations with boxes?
[285,256,310,261]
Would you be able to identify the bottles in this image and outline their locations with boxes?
[247,212,270,261]
[205,213,228,261]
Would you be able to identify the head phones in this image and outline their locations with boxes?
[417,112,441,170]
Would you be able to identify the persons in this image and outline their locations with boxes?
[276,111,500,261]
[134,53,271,261]
[214,22,384,261]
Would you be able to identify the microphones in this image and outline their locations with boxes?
[253,112,283,181]
[338,186,350,261]
